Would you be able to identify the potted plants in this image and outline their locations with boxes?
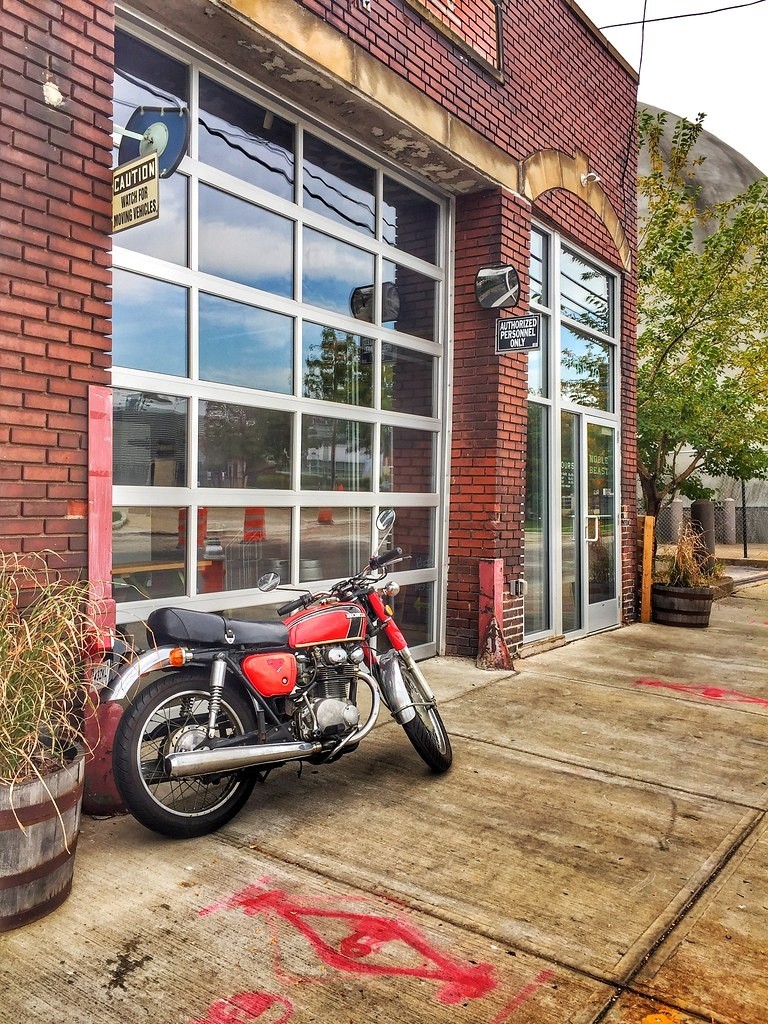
[2,549,156,934]
[649,519,723,629]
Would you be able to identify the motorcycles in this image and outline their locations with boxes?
[92,510,454,841]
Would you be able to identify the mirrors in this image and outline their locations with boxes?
[474,264,521,310]
[348,280,401,324]
